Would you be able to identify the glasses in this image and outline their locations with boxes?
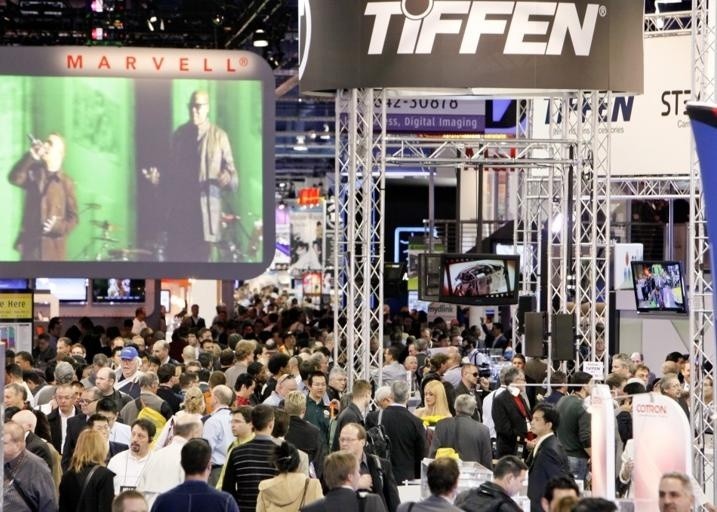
[188,103,208,110]
[80,398,98,405]
[280,374,295,384]
[632,360,640,364]
[338,437,357,441]
[472,372,477,377]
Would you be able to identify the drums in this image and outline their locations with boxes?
[108,249,154,262]
[130,241,174,263]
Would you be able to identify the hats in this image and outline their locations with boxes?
[120,346,138,360]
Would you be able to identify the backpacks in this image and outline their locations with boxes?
[365,424,392,461]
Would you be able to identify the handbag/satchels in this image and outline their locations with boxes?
[136,407,167,451]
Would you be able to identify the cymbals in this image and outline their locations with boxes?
[84,203,100,210]
[90,220,125,232]
[90,236,120,244]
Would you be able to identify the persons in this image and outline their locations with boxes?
[633,263,684,309]
[136,89,241,264]
[9,129,80,262]
[1,283,715,512]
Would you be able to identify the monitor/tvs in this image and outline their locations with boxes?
[90,278,146,305]
[440,253,520,305]
[0,279,29,290]
[631,261,689,315]
[418,253,440,301]
[33,278,88,306]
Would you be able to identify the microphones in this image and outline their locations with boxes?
[27,132,41,152]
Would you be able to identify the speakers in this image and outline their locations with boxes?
[551,314,576,360]
[524,311,547,358]
